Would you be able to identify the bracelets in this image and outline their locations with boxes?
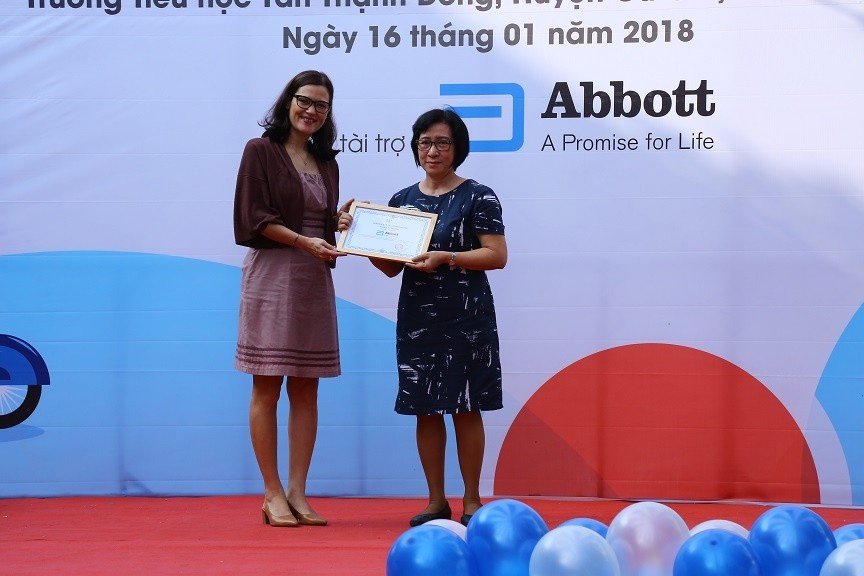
[293,232,300,247]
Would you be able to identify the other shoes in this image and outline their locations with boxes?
[410,500,451,527]
[460,503,483,527]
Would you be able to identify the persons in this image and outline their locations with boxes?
[357,108,508,525]
[233,68,370,527]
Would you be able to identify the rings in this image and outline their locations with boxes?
[329,256,332,262]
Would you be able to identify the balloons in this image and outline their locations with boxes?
[382,497,864,576]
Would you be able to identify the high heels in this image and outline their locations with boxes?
[262,501,300,528]
[287,501,328,525]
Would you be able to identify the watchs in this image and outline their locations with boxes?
[449,250,456,271]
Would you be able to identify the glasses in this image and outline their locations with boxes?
[415,139,455,152]
[293,95,331,113]
